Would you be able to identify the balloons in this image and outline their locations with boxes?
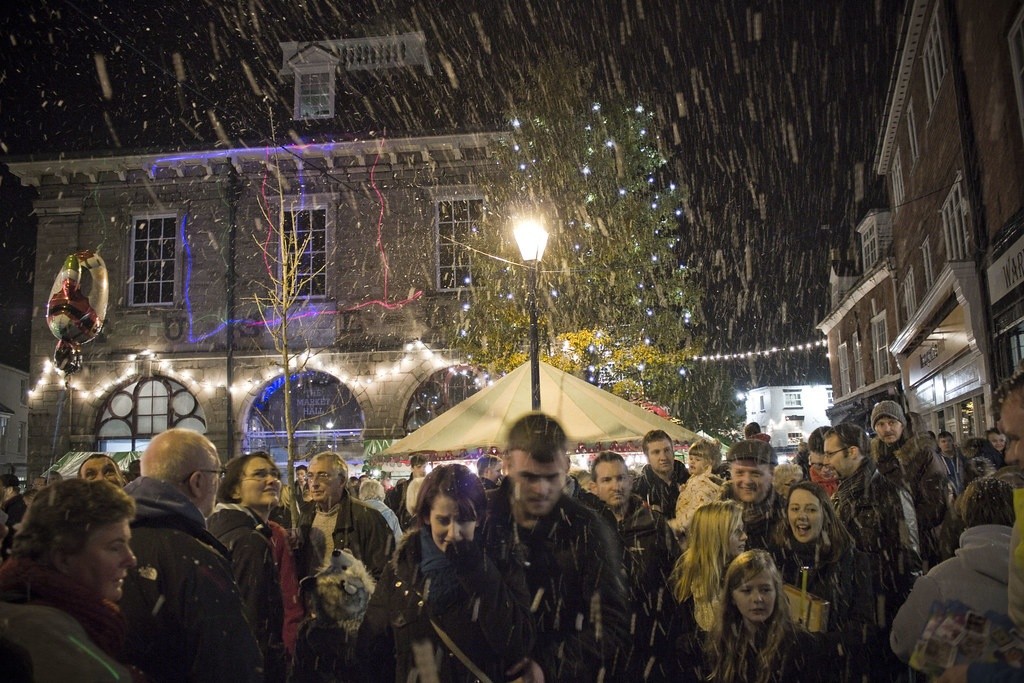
[47,250,108,378]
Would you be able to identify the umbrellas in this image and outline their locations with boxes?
[375,359,704,450]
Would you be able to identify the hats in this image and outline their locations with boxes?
[0,474,20,487]
[871,400,907,431]
[724,439,778,466]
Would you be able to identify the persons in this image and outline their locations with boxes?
[0,371,1024,683]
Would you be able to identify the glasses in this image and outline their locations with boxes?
[808,456,834,471]
[824,446,849,457]
[304,472,339,480]
[182,468,226,485]
[238,471,281,482]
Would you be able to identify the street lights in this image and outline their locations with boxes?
[512,216,549,411]
